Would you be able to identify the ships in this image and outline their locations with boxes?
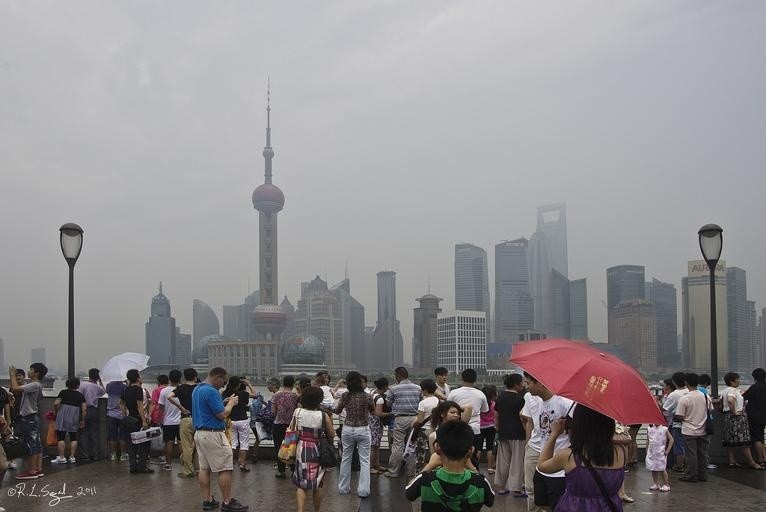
[60,374,68,382]
[50,375,58,381]
[81,376,89,381]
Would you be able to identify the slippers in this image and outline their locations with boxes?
[500,491,508,494]
[514,493,528,497]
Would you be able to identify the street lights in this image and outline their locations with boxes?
[58,222,84,383]
[697,222,724,393]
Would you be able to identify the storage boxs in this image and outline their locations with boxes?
[130,427,162,445]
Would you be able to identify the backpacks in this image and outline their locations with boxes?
[375,395,392,412]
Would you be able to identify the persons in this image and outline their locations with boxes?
[0,363,764,512]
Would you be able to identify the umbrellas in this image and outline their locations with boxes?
[100,352,151,383]
[506,339,670,429]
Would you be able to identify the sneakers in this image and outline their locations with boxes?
[621,495,634,502]
[10,453,250,479]
[371,464,397,478]
[488,468,495,473]
[203,496,250,510]
[650,459,765,492]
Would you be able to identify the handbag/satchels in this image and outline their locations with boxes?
[123,416,139,430]
[277,430,299,464]
[706,412,714,435]
[319,429,338,467]
[47,422,57,446]
